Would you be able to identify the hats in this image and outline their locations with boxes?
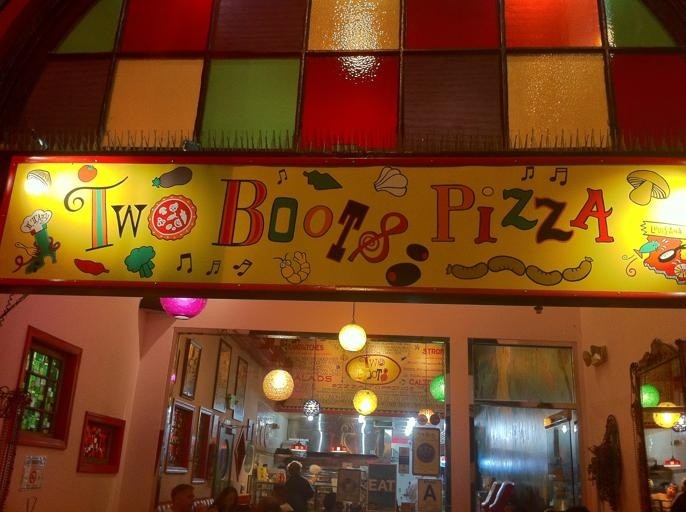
[288,462,302,474]
[324,493,336,508]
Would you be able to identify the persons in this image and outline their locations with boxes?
[670,478,686,512]
[286,459,314,512]
[256,496,280,512]
[650,483,677,512]
[207,487,238,512]
[164,484,195,512]
[273,486,294,512]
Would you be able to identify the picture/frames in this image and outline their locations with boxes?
[77,410,126,474]
[165,338,254,498]
[336,426,442,512]
[18,455,47,490]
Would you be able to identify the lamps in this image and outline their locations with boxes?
[429,375,446,403]
[338,301,367,353]
[263,369,295,402]
[24,169,51,197]
[419,408,435,422]
[159,296,207,320]
[353,389,378,415]
[304,398,321,423]
[640,384,686,467]
